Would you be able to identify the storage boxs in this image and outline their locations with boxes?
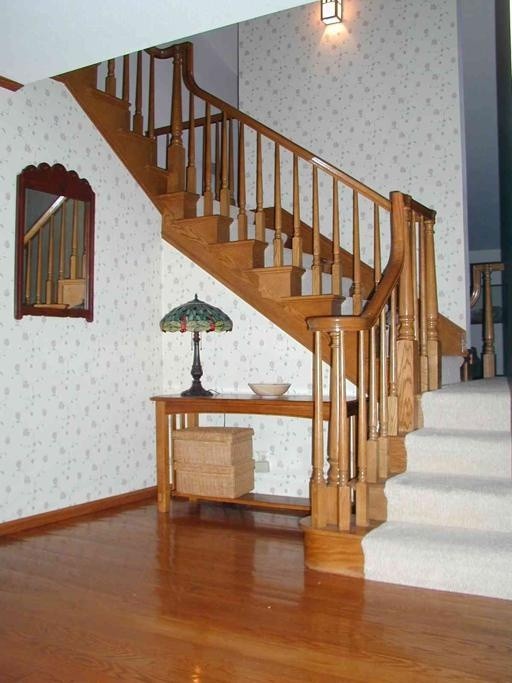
[171,426,255,499]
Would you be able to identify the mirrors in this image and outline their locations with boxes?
[16,162,95,322]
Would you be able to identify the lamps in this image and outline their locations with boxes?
[160,293,233,397]
[321,0,343,25]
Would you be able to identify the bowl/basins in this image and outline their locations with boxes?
[248,382,292,398]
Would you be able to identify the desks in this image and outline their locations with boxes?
[150,392,369,513]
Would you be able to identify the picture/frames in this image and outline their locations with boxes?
[470,284,505,324]
